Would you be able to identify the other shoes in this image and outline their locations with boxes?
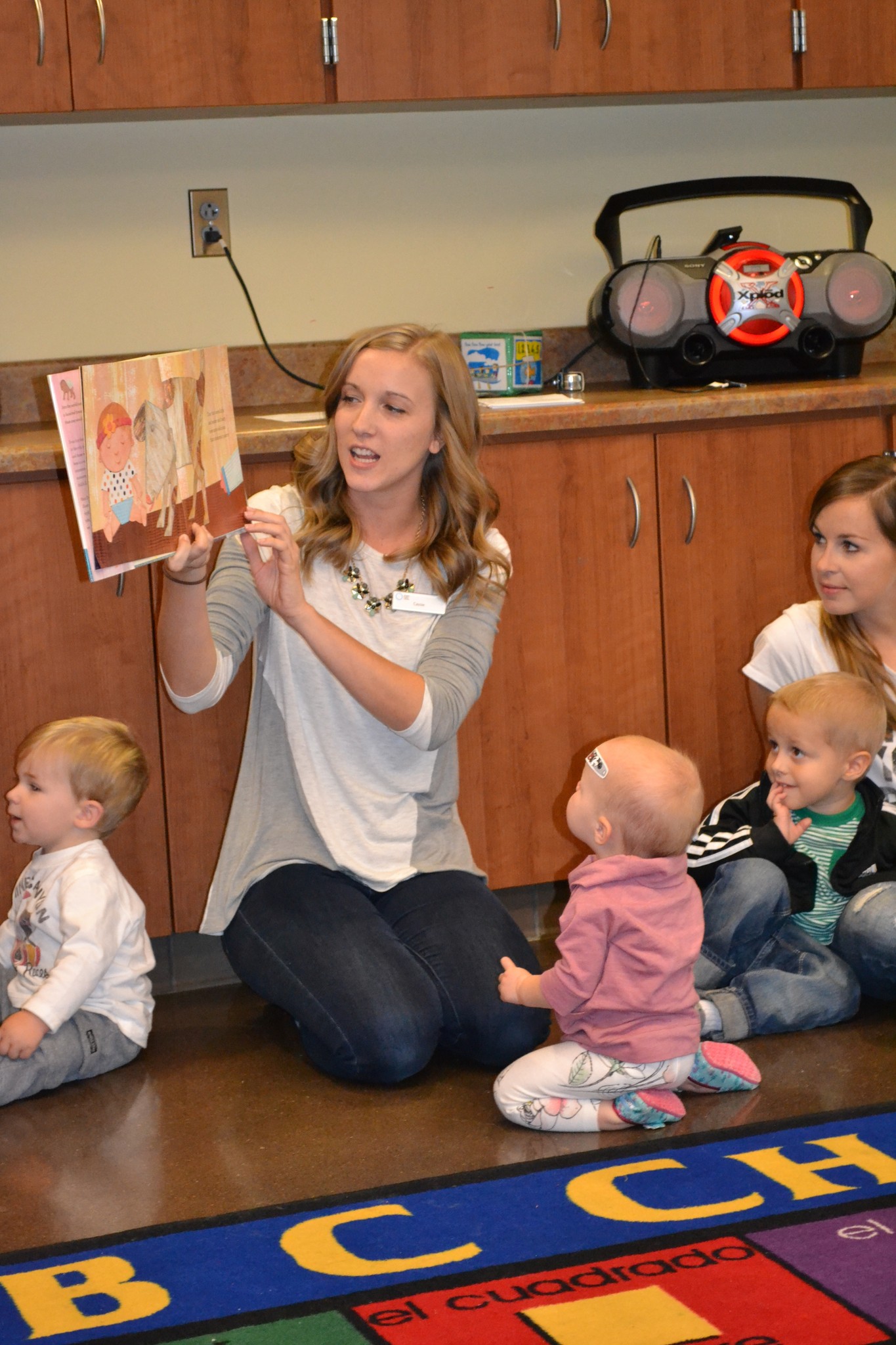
[614,1088,686,1130]
[688,1041,761,1092]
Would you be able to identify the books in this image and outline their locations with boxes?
[47,343,254,583]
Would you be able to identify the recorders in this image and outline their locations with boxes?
[589,173,896,387]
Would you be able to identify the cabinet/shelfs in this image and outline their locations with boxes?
[1,0,326,126]
[0,359,894,1000]
[326,1,797,116]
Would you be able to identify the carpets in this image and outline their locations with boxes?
[2,1097,895,1345]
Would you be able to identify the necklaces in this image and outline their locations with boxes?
[341,489,426,617]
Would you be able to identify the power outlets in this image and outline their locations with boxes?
[188,188,232,258]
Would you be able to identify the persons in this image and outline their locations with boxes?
[742,449,896,1008]
[681,670,896,1044]
[155,321,552,1087]
[0,715,156,1108]
[493,734,762,1133]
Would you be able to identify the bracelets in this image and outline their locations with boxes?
[161,564,208,585]
